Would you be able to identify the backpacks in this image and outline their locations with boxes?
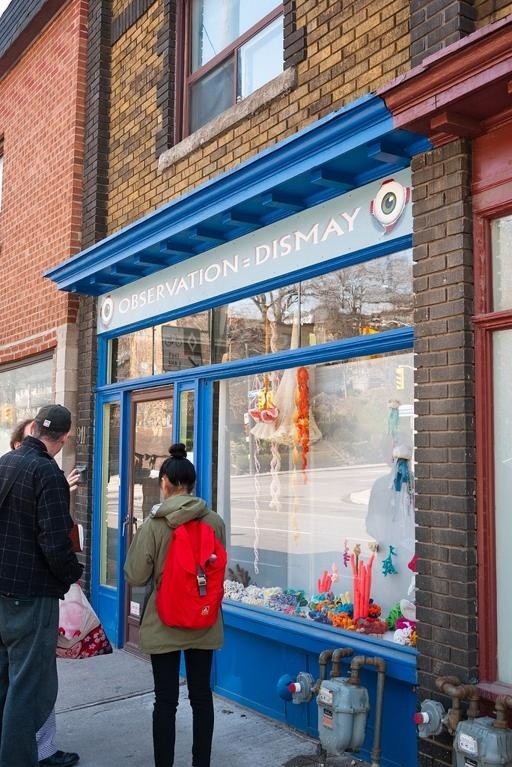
[156,518,227,631]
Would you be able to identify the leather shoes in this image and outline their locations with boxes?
[39,751,81,764]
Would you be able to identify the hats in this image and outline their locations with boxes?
[34,405,71,433]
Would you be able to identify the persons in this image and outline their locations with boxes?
[2,401,86,767]
[122,441,227,767]
[363,436,416,617]
[8,419,81,767]
